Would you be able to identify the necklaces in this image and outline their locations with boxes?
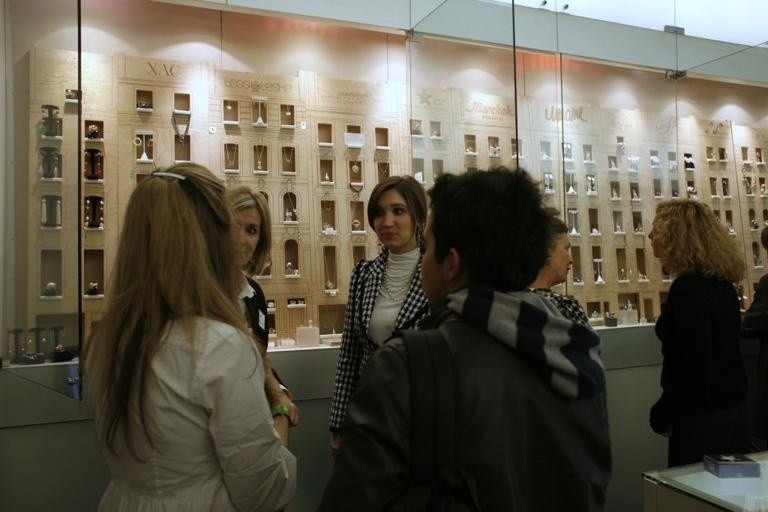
[349,149,365,175]
[324,303,344,335]
[322,247,340,290]
[283,147,294,164]
[286,192,301,213]
[284,105,293,118]
[376,150,390,176]
[255,144,267,170]
[171,111,192,145]
[322,185,336,214]
[381,257,418,303]
[225,145,241,167]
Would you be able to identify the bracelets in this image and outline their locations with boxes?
[270,402,294,415]
[273,412,292,428]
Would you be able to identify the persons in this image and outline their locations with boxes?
[77,160,296,512]
[218,183,293,404]
[317,163,613,512]
[329,174,431,466]
[744,224,768,454]
[647,200,748,469]
[532,212,591,325]
